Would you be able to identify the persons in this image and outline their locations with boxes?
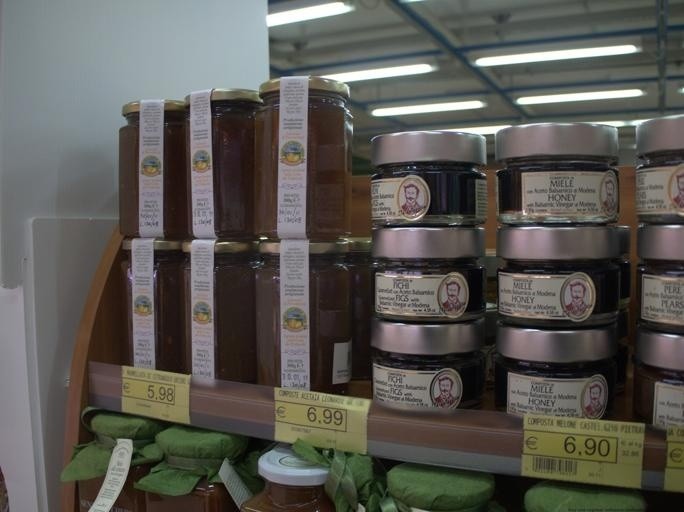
[585,383,603,416]
[400,182,425,216]
[603,180,616,213]
[433,376,456,408]
[565,281,587,317]
[672,174,684,209]
[442,281,462,311]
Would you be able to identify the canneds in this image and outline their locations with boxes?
[78,75,684,512]
